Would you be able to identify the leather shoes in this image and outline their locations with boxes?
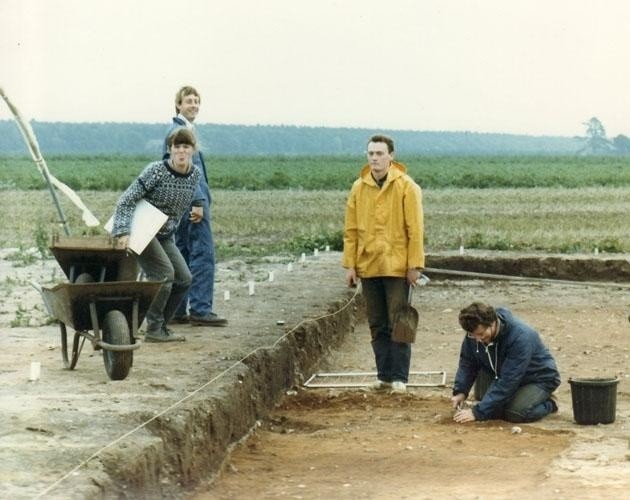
[167,308,228,326]
[145,327,185,342]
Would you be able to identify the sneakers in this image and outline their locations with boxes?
[360,379,408,394]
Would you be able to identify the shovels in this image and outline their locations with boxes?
[392,267,425,343]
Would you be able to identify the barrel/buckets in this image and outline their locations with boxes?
[567,374,620,424]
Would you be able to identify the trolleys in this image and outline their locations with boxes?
[28,277,168,381]
[47,210,140,285]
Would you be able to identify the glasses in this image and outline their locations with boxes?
[467,330,489,340]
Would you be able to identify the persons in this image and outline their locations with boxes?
[447,301,561,424]
[337,133,426,394]
[110,127,203,342]
[162,85,230,327]
